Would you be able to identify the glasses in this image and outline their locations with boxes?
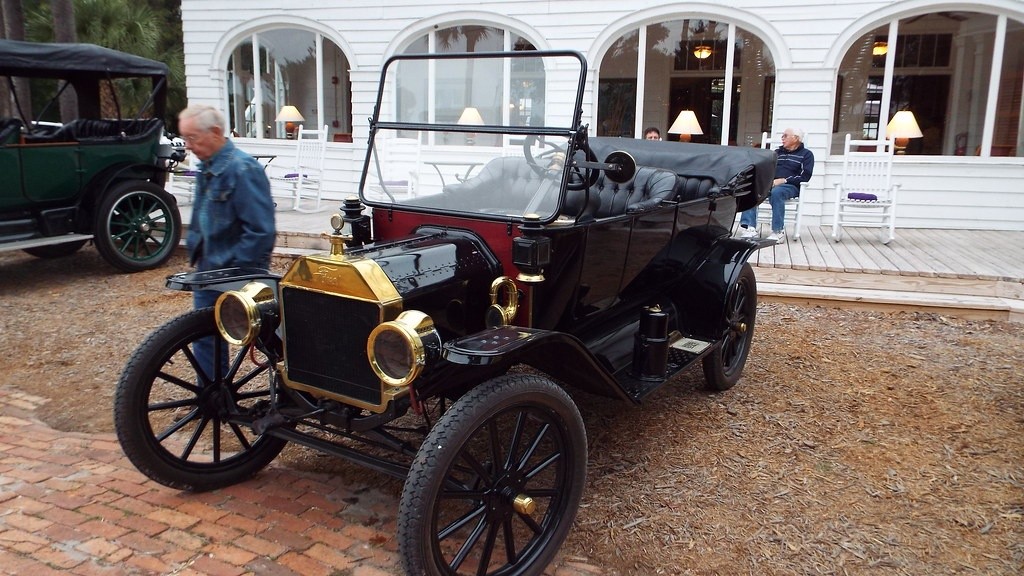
[781,134,798,140]
[179,128,210,145]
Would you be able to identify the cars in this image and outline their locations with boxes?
[114,50,779,576]
[0,37,187,274]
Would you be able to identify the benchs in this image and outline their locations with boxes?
[556,139,776,212]
[0,117,22,144]
[442,155,677,224]
[51,119,161,144]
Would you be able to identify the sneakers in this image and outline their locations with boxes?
[740,226,758,241]
[766,229,785,244]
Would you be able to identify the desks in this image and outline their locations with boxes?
[249,154,276,170]
[424,161,484,193]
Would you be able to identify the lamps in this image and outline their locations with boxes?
[667,110,704,143]
[275,106,305,139]
[887,110,923,155]
[456,108,485,147]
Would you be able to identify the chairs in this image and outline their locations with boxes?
[270,124,329,214]
[975,144,1013,156]
[169,146,200,205]
[752,132,808,241]
[366,138,419,201]
[832,133,902,244]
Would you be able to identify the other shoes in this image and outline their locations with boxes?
[173,408,203,422]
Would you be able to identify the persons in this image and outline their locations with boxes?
[643,127,660,141]
[739,127,815,243]
[171,105,278,425]
